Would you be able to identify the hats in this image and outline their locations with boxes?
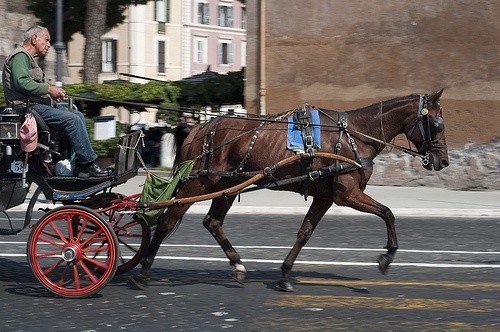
[19,114,37,152]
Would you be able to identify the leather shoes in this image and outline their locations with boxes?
[78,161,109,177]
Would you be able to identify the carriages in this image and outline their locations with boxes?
[0,87,449,298]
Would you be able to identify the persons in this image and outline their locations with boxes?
[2,26,113,178]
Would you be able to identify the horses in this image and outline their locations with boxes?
[129,87,451,292]
